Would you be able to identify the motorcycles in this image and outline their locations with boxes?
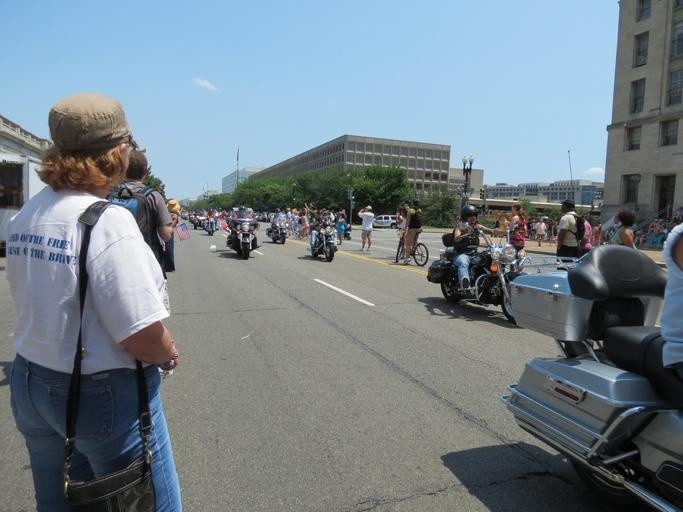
[264,218,288,245]
[506,243,682,510]
[308,221,337,263]
[225,209,257,260]
[426,223,530,326]
[181,210,223,237]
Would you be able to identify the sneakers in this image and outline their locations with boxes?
[463,278,472,297]
[399,256,411,264]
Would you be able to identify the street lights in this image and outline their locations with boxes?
[462,155,474,208]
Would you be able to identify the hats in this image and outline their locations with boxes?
[560,199,576,205]
[49,93,131,151]
[412,198,420,206]
[365,205,372,210]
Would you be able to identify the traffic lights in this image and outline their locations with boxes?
[351,191,356,202]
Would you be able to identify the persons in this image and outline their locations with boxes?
[451,205,506,298]
[495,203,527,272]
[396,199,423,265]
[271,203,346,245]
[163,199,180,279]
[357,205,374,251]
[527,217,558,247]
[661,222,683,377]
[556,199,636,272]
[106,150,172,279]
[189,206,261,250]
[634,207,683,250]
[5,94,182,512]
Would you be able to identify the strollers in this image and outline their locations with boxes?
[343,224,351,240]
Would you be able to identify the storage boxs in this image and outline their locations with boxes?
[511,269,597,343]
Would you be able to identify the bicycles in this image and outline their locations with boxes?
[394,228,429,268]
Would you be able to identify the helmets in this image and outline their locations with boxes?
[460,205,478,217]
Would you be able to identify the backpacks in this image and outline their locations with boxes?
[562,210,592,242]
[105,187,164,267]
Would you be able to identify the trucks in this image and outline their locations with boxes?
[0,154,49,252]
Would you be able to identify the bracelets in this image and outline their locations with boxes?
[160,349,179,368]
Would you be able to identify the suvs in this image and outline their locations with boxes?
[372,215,396,228]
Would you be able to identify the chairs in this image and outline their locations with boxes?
[569,243,682,409]
[441,232,464,257]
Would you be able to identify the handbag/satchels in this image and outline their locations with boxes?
[67,455,156,512]
